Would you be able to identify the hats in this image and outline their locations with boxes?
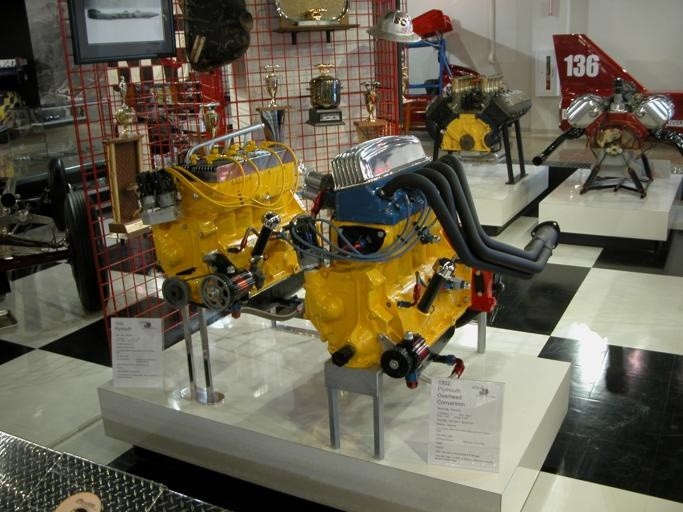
[366,10,422,45]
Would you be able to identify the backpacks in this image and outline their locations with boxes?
[184,0,253,72]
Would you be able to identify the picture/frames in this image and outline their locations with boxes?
[68,0,177,65]
